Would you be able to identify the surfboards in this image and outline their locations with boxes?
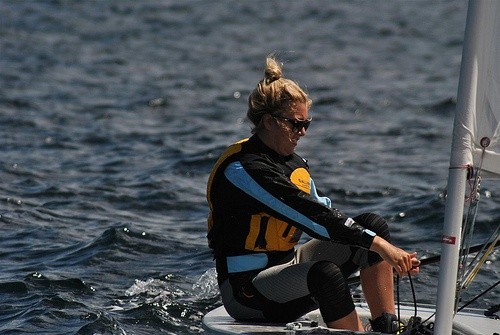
[203,302,500,335]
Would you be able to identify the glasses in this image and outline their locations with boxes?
[268,112,311,134]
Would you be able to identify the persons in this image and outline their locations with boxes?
[206,57,421,334]
